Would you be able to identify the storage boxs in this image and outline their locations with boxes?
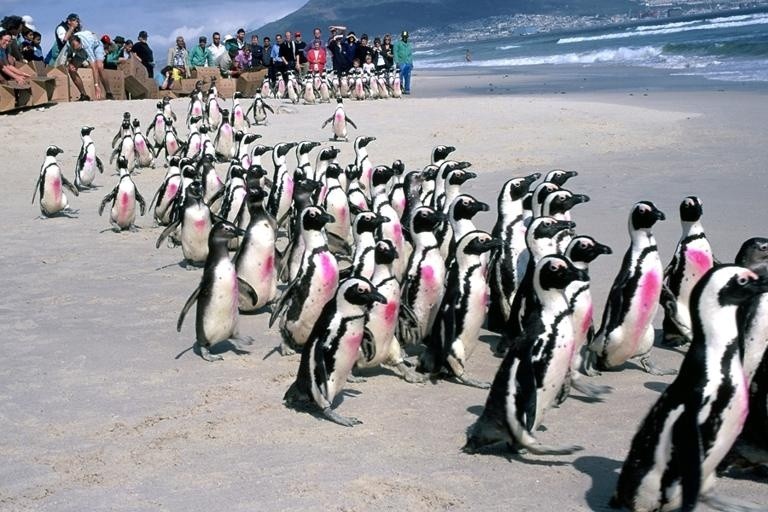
[0,52,268,112]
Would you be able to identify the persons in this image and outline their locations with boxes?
[0,15,44,83]
[162,28,327,89]
[44,14,155,99]
[327,25,413,94]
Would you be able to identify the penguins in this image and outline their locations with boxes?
[31,143,80,222]
[462,168,767,512]
[98,56,509,428]
[74,126,104,191]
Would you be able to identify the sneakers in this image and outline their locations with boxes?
[78,94,90,101]
[106,92,113,100]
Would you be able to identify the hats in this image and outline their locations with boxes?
[400,31,408,37]
[199,36,207,43]
[295,32,301,37]
[21,15,35,32]
[67,13,80,21]
[112,36,127,44]
[139,30,150,38]
